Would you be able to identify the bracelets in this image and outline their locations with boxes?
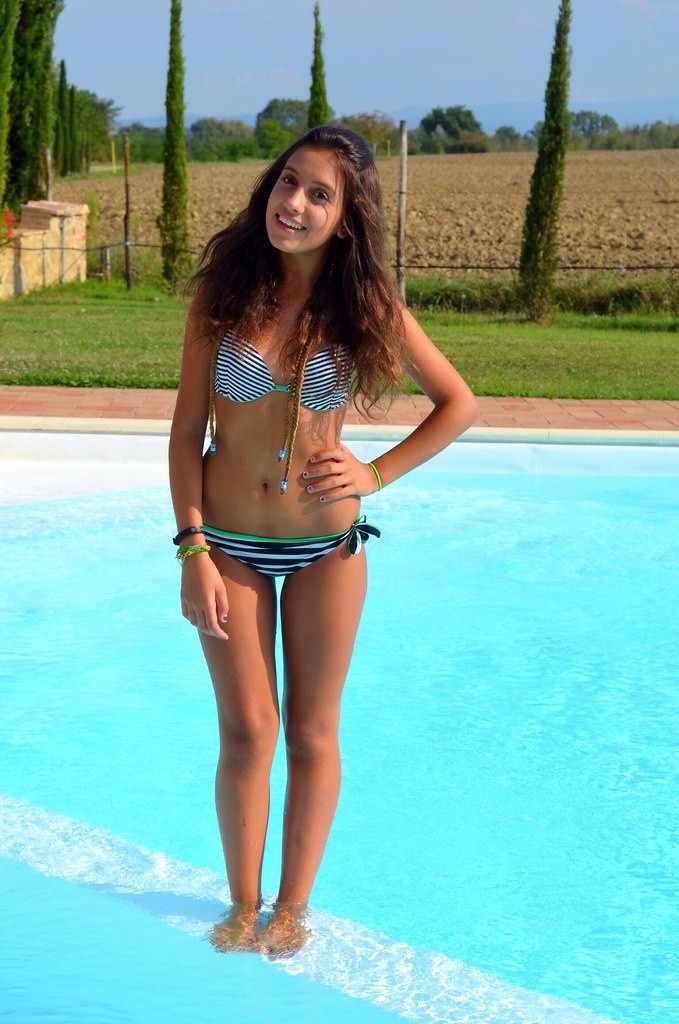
[175,542,211,566]
[173,523,203,544]
[368,462,382,490]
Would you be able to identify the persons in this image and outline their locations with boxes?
[168,128,478,904]
